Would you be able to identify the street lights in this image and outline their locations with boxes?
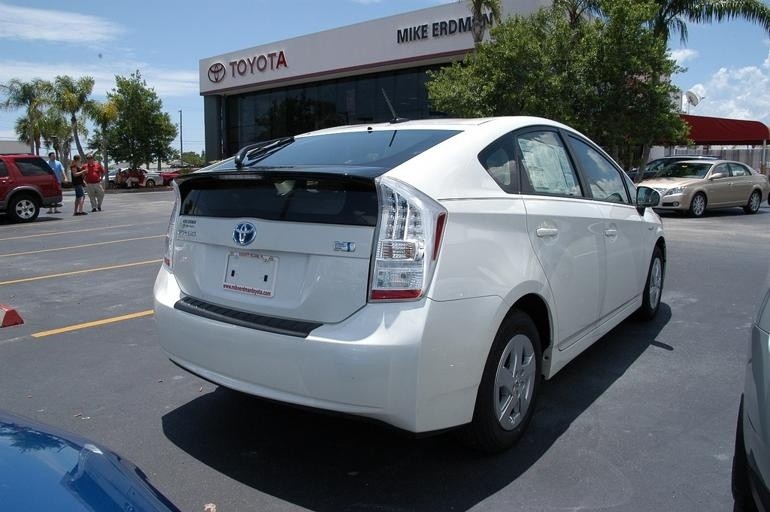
[178,109,183,166]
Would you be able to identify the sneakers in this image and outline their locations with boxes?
[46,207,101,215]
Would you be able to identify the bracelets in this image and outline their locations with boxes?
[80,172,83,177]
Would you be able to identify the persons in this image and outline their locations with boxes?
[45,152,68,213]
[70,155,88,215]
[117,166,145,189]
[80,155,105,212]
